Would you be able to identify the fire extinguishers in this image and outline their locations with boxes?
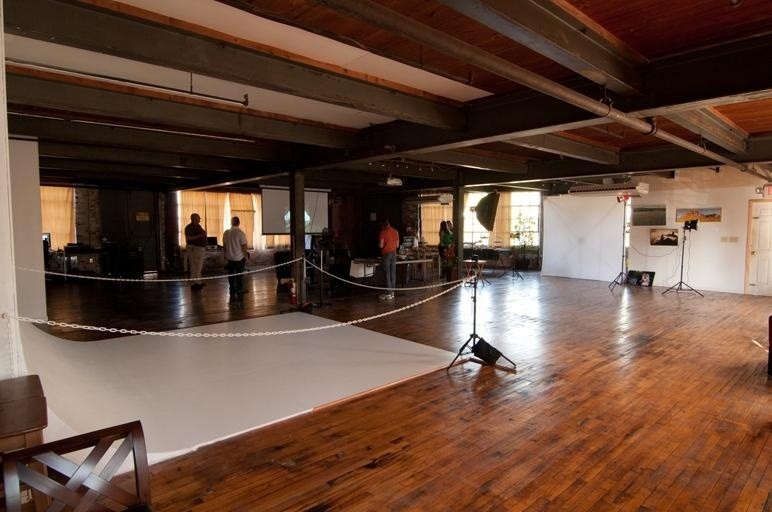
[288,279,297,304]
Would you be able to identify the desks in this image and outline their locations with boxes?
[461,260,491,288]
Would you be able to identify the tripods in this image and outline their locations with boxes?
[608,200,639,293]
[446,270,516,372]
[662,225,708,298]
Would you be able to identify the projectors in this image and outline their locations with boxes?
[377,176,403,187]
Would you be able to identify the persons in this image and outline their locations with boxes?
[223,217,248,294]
[378,220,400,299]
[185,213,208,290]
[447,221,454,231]
[439,221,453,278]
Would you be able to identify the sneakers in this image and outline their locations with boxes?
[191,283,206,290]
[379,291,395,300]
[238,288,248,295]
